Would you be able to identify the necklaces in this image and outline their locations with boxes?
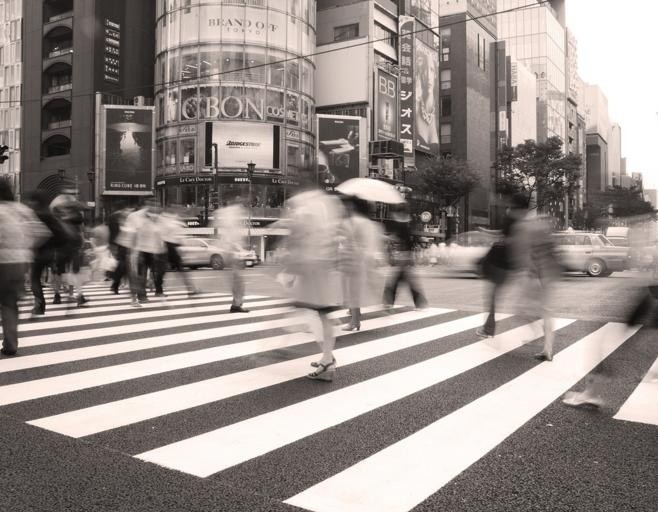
[420,99,436,125]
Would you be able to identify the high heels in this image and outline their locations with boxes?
[305,356,335,382]
[341,321,362,332]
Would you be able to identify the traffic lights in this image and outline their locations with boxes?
[0,143,10,165]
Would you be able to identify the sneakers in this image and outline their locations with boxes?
[229,305,249,314]
[1,283,205,357]
[533,349,553,361]
[474,326,495,340]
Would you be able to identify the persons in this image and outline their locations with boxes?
[0,179,658,411]
[416,57,439,154]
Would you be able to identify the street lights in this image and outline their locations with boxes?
[246,159,258,251]
[87,168,95,202]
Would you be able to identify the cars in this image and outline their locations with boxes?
[428,222,656,277]
[174,235,259,270]
